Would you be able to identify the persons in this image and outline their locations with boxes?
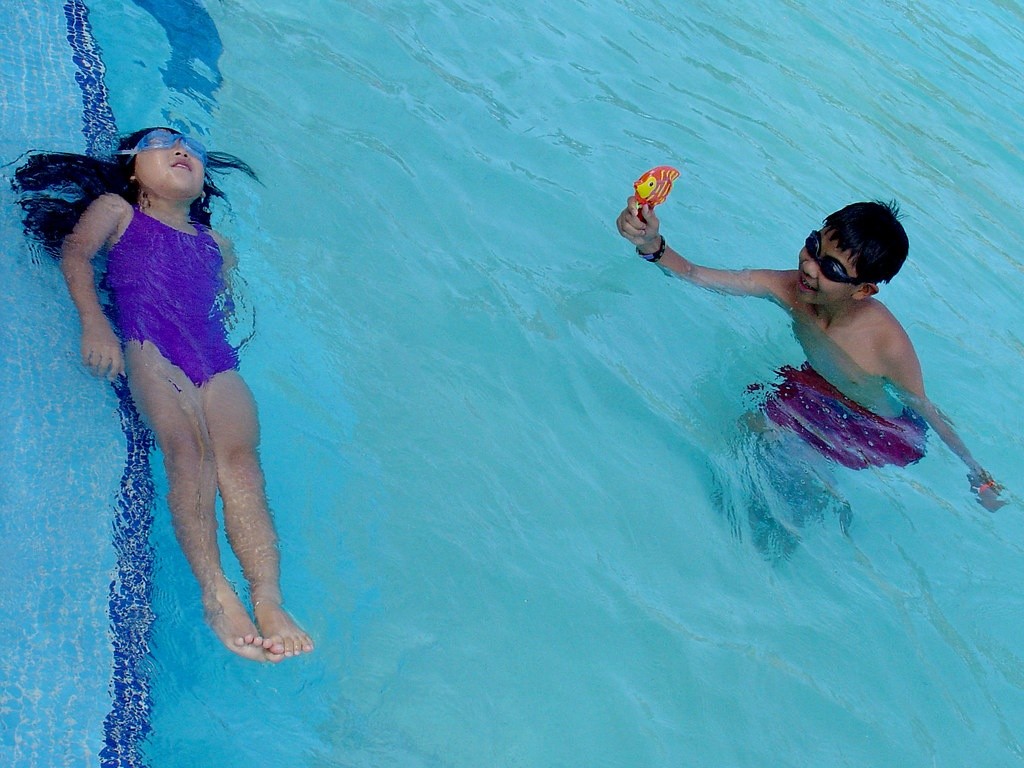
[63,127,314,662]
[617,196,926,397]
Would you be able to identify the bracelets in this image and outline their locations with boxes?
[636,234,666,263]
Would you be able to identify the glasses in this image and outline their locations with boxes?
[805,230,861,286]
[110,129,207,169]
